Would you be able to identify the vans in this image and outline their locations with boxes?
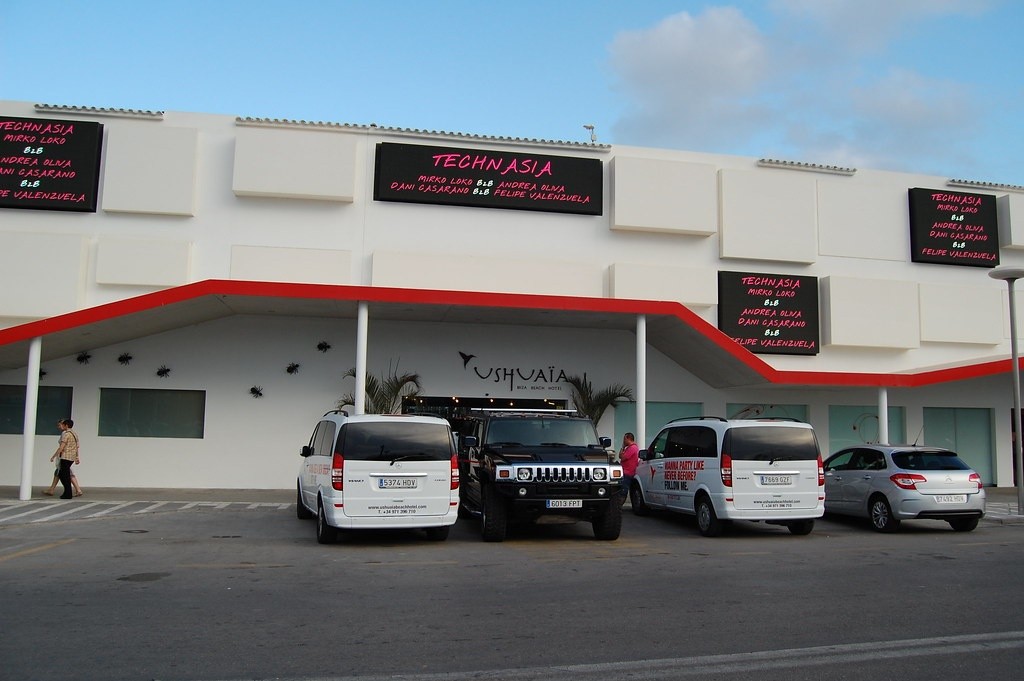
[630,416,827,536]
[295,408,461,545]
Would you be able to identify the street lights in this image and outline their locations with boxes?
[986,266,1023,516]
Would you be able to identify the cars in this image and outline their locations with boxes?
[824,444,986,534]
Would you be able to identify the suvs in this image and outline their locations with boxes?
[456,407,625,543]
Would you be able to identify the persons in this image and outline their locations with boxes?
[41,419,83,499]
[619,432,639,509]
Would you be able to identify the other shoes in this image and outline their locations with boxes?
[42,490,54,496]
[60,493,72,499]
[73,490,83,497]
